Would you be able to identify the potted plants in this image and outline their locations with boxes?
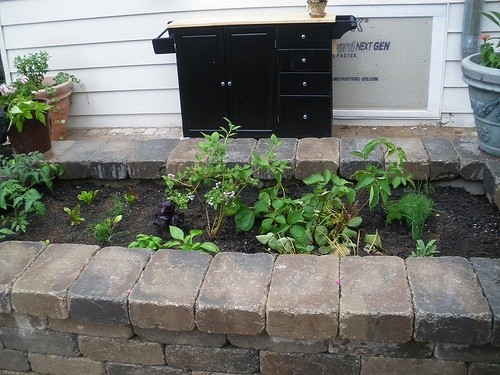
[11,50,84,140]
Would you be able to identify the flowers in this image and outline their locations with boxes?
[477,12,500,69]
[0,73,50,128]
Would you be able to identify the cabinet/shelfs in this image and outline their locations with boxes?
[153,14,360,141]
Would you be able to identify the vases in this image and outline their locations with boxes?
[460,52,500,156]
[8,98,56,156]
